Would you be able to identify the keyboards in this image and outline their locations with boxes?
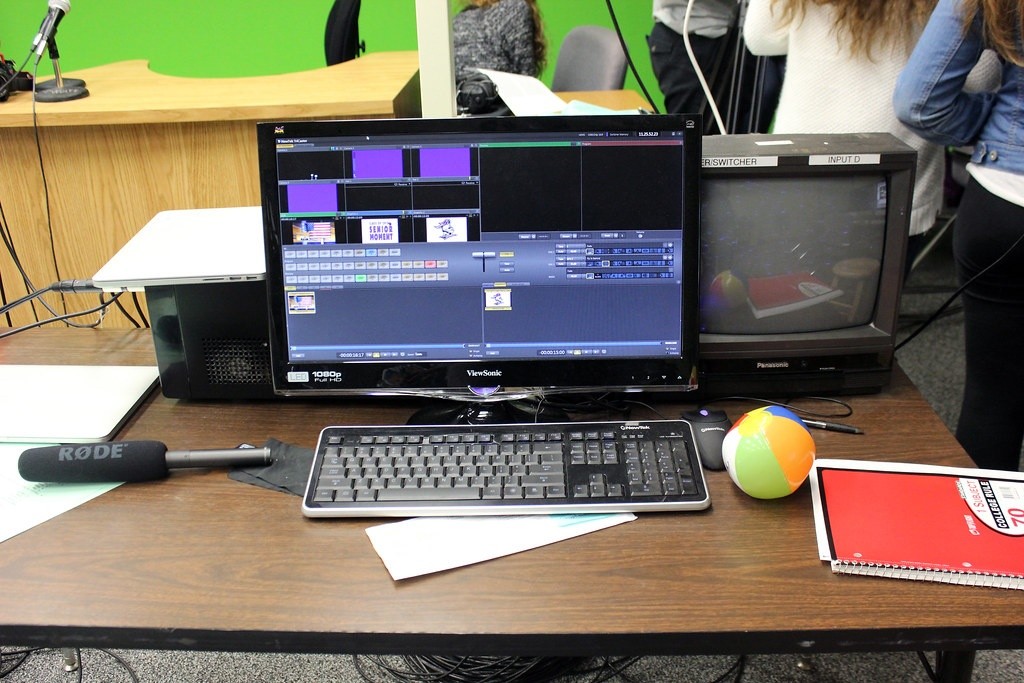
[301,419,712,518]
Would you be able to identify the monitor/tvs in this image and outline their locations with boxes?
[681,131,919,407]
[257,113,704,425]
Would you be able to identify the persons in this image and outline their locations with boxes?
[891,0,1023,469]
[645,0,1004,284]
[449,0,547,88]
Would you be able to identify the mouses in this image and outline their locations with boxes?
[682,407,736,472]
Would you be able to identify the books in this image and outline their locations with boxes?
[809,458,1024,592]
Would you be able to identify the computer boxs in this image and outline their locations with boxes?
[141,280,461,408]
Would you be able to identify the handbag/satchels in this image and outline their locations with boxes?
[455,72,500,117]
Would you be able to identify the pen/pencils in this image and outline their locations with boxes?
[801,417,867,434]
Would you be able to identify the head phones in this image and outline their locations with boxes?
[455,72,502,114]
[0,61,34,103]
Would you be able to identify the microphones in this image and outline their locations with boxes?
[19,439,274,484]
[29,0,71,65]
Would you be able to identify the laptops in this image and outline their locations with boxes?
[94,206,268,290]
